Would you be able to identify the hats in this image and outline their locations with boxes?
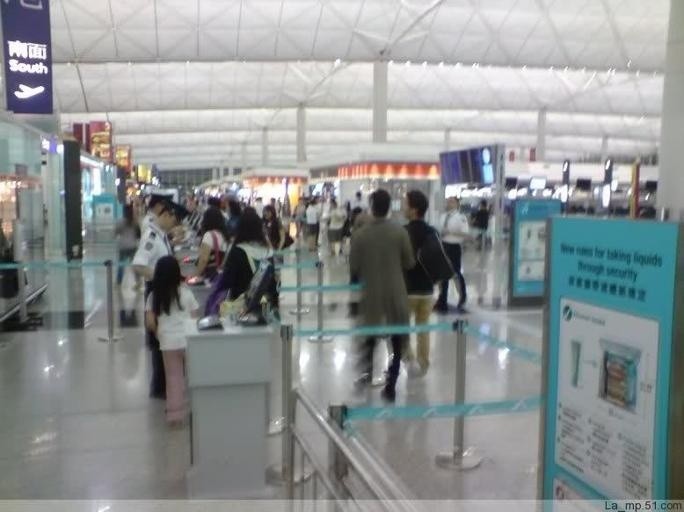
[150,194,192,224]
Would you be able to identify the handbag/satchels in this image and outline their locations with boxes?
[274,232,294,251]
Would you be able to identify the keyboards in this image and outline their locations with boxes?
[197,312,222,331]
[186,276,204,285]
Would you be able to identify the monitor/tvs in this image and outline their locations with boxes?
[187,209,238,278]
[240,259,274,326]
